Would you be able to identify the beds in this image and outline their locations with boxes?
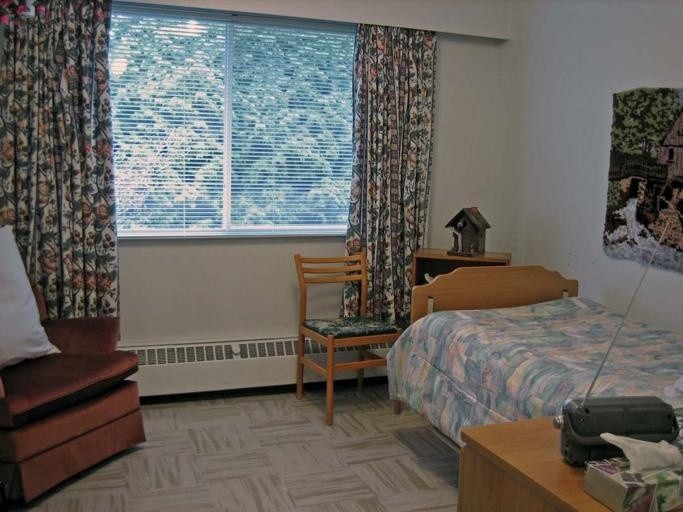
[385,264,683,452]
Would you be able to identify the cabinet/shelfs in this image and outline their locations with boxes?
[410,247,512,288]
[456,414,617,512]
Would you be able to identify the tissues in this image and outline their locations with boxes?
[584,432,682,510]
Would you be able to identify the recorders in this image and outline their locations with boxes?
[552,393,678,467]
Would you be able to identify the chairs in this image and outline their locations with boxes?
[0,270,146,512]
[293,251,403,426]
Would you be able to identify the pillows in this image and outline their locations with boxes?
[0,225,62,369]
